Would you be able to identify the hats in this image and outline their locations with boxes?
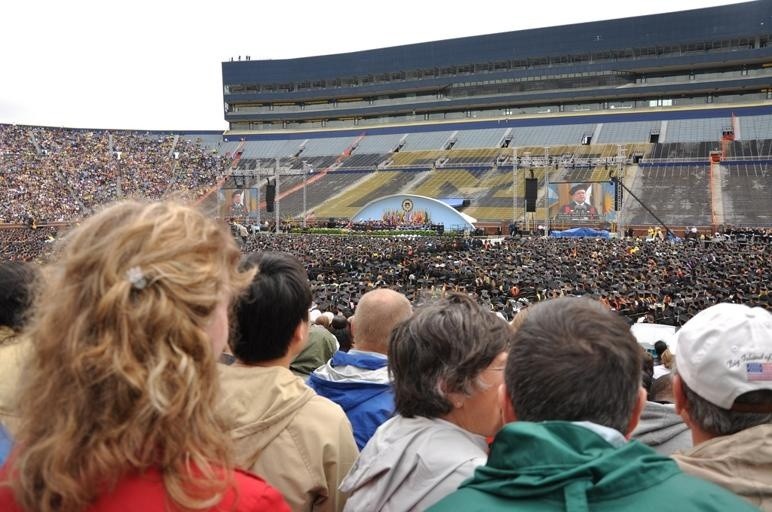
[232,190,242,198]
[669,303,772,413]
[333,315,346,328]
[569,184,587,195]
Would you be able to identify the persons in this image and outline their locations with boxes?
[664,304,772,511]
[340,292,511,512]
[0,199,298,511]
[425,293,765,511]
[211,252,363,512]
[1,124,772,465]
